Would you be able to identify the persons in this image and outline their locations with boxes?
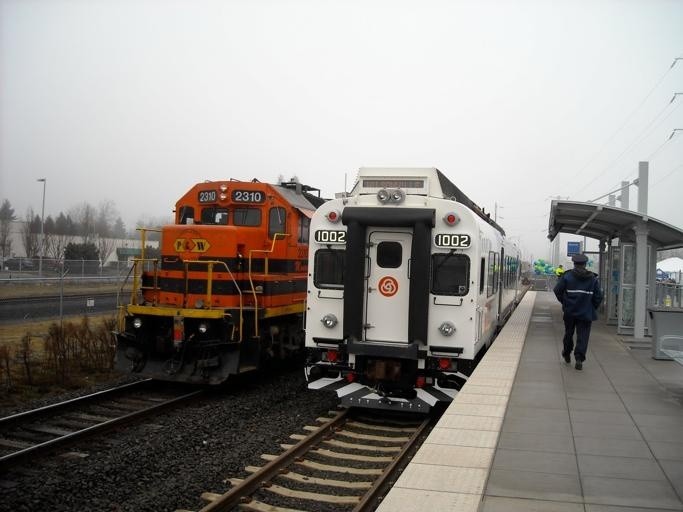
[555,265,566,279]
[656,276,678,288]
[552,253,604,371]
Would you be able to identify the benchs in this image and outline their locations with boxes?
[660,335,683,365]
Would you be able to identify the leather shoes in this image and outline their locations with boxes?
[562,348,571,363]
[575,361,583,370]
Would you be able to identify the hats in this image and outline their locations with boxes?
[572,254,589,263]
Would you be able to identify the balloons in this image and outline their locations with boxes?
[532,257,555,275]
